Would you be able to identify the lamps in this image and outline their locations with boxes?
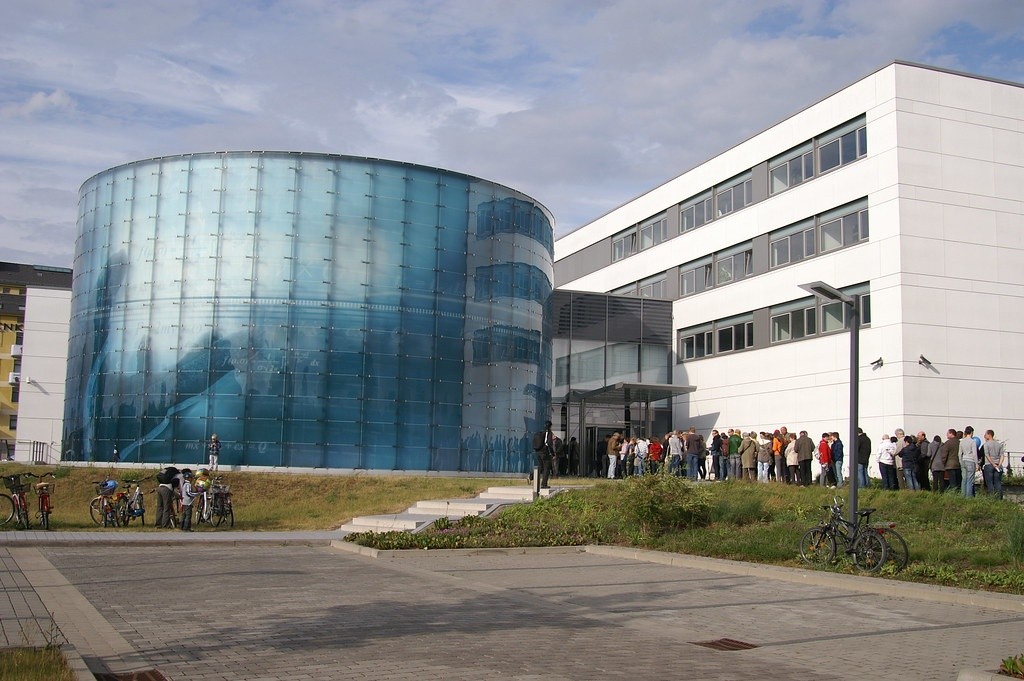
[26,377,30,384]
[919,355,932,365]
[870,357,883,367]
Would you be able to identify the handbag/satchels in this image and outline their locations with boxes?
[928,460,933,469]
[769,457,773,465]
[706,450,709,455]
[974,467,984,484]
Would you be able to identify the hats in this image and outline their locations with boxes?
[774,430,779,434]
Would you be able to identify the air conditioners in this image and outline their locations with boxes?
[8,371,20,384]
[11,344,23,356]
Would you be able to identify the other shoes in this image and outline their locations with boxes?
[830,485,836,490]
[540,485,551,489]
[527,478,531,485]
[181,527,195,533]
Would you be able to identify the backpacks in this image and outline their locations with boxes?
[157,467,180,484]
[775,436,787,457]
[814,442,826,460]
[533,431,548,450]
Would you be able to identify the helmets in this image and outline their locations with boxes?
[184,472,194,478]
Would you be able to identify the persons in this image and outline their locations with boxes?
[597,426,844,489]
[550,436,579,480]
[856,427,871,487]
[207,434,222,471]
[527,421,553,489]
[876,426,1004,500]
[155,467,199,532]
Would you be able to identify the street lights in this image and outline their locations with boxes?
[797,280,861,562]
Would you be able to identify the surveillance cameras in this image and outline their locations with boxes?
[920,355,932,364]
[870,357,882,365]
[26,377,30,384]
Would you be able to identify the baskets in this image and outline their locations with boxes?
[95,486,115,495]
[212,484,231,495]
[33,483,55,495]
[3,475,20,489]
[10,483,31,494]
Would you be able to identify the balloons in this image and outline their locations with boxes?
[195,468,210,493]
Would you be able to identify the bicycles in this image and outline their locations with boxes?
[0,469,234,531]
[799,494,910,577]
